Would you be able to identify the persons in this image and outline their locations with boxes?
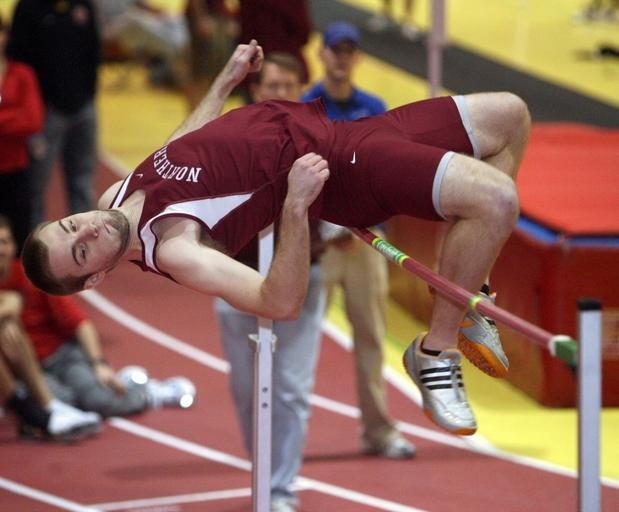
[20,41,530,436]
[210,53,353,511]
[297,24,417,459]
[2,1,196,440]
[184,2,313,110]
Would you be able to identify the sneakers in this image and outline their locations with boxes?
[15,406,101,440]
[118,364,195,406]
[357,424,416,461]
[404,331,477,435]
[428,280,509,378]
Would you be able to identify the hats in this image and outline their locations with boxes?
[324,21,360,48]
